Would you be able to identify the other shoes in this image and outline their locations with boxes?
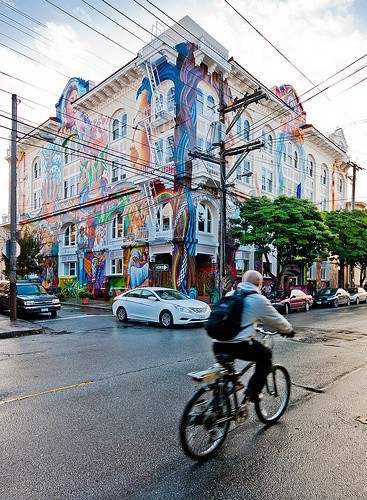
[243,393,266,402]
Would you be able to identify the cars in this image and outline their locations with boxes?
[314,288,351,307]
[112,287,211,328]
[0,282,62,321]
[346,287,367,304]
[266,289,314,314]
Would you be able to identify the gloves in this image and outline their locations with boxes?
[282,331,297,340]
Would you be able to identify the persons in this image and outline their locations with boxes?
[206,269,293,403]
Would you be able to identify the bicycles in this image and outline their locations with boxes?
[179,325,296,461]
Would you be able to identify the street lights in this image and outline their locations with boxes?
[9,132,63,321]
[219,172,253,300]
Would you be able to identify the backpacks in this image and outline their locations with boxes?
[204,288,261,341]
